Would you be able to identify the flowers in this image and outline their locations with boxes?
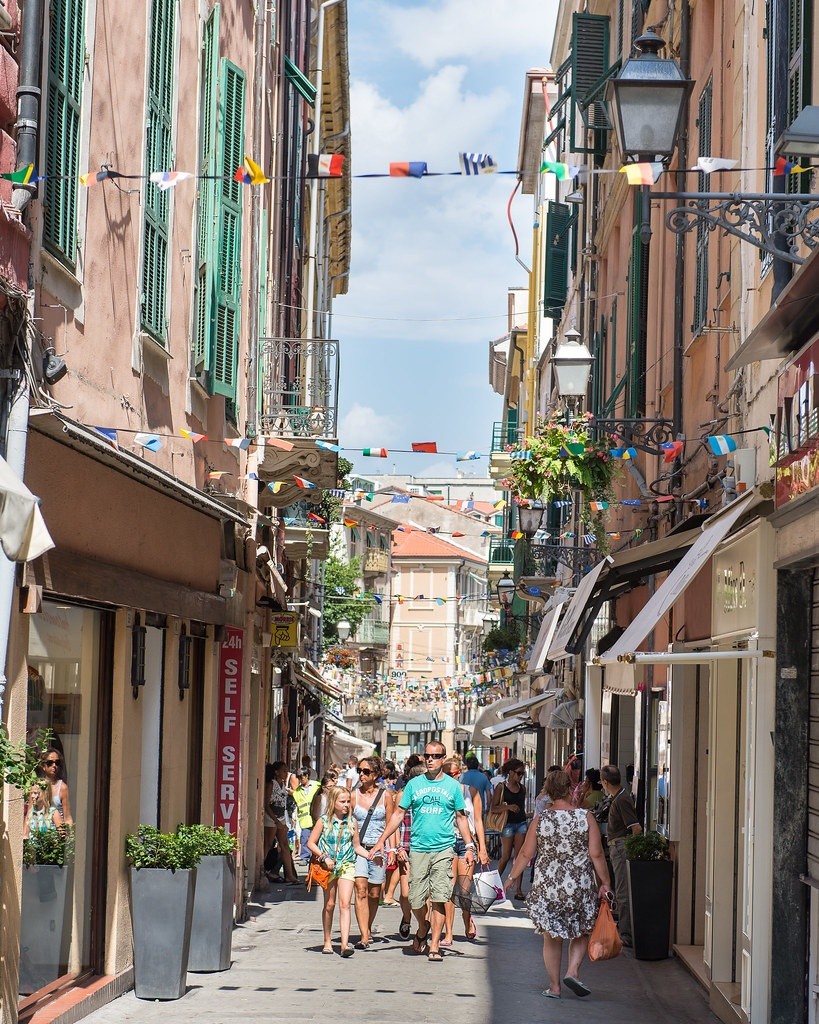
[324,646,357,669]
[500,410,627,503]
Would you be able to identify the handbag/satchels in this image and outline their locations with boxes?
[587,898,623,961]
[485,782,509,833]
[473,862,507,906]
[305,855,332,893]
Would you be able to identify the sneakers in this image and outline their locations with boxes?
[399,912,412,940]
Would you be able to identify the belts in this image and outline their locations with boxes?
[362,844,374,850]
[608,836,629,845]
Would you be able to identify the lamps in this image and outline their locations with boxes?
[304,615,352,657]
[298,680,321,716]
[564,192,584,204]
[773,105,819,159]
[599,25,819,265]
[43,350,69,386]
[258,596,284,612]
[518,503,604,575]
[495,568,542,630]
[595,625,627,656]
[287,600,322,619]
[546,324,674,456]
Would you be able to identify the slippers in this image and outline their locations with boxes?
[564,976,591,997]
[441,938,453,946]
[429,951,443,961]
[542,989,561,1000]
[413,920,431,953]
[463,913,476,939]
[287,881,302,885]
[382,900,401,908]
[322,946,333,953]
[340,947,354,957]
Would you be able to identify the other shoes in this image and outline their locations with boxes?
[355,941,371,949]
[368,936,374,943]
[268,876,285,883]
[515,894,527,901]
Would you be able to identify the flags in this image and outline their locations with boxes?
[314,581,508,603]
[205,470,707,507]
[265,510,643,541]
[1,156,812,191]
[97,425,737,463]
[328,647,520,713]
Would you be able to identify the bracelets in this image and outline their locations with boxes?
[508,874,519,881]
[318,852,328,863]
[465,843,474,849]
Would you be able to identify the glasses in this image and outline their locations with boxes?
[424,753,446,760]
[513,770,526,776]
[357,767,375,775]
[447,770,460,777]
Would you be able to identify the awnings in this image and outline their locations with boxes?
[546,526,702,661]
[591,494,772,664]
[296,670,343,707]
[525,581,628,674]
[471,690,556,747]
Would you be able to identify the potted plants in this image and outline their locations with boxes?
[18,822,75,995]
[176,822,241,974]
[623,831,675,962]
[481,618,521,653]
[124,824,202,1002]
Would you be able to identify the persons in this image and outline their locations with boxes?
[491,760,642,946]
[264,756,322,887]
[308,741,493,960]
[23,749,72,839]
[504,770,610,997]
